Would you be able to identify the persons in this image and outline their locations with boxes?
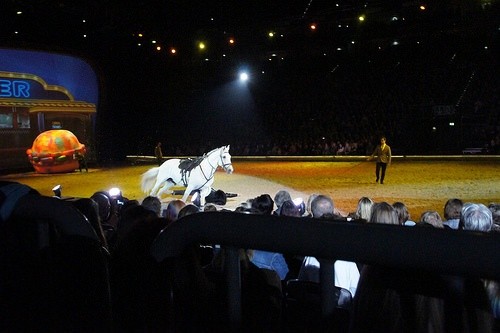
[304,194,318,217]
[487,204,500,231]
[366,137,391,184]
[242,209,289,280]
[0,178,241,333]
[392,202,410,226]
[293,198,305,216]
[125,37,499,155]
[280,200,299,217]
[442,198,464,229]
[273,190,292,216]
[355,197,374,222]
[421,212,444,230]
[311,195,334,220]
[298,213,361,299]
[369,202,399,225]
[458,203,493,231]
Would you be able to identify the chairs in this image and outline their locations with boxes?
[143,211,500,333]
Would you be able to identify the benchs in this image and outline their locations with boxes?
[0,181,112,333]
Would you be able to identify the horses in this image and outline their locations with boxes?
[140,144,234,206]
